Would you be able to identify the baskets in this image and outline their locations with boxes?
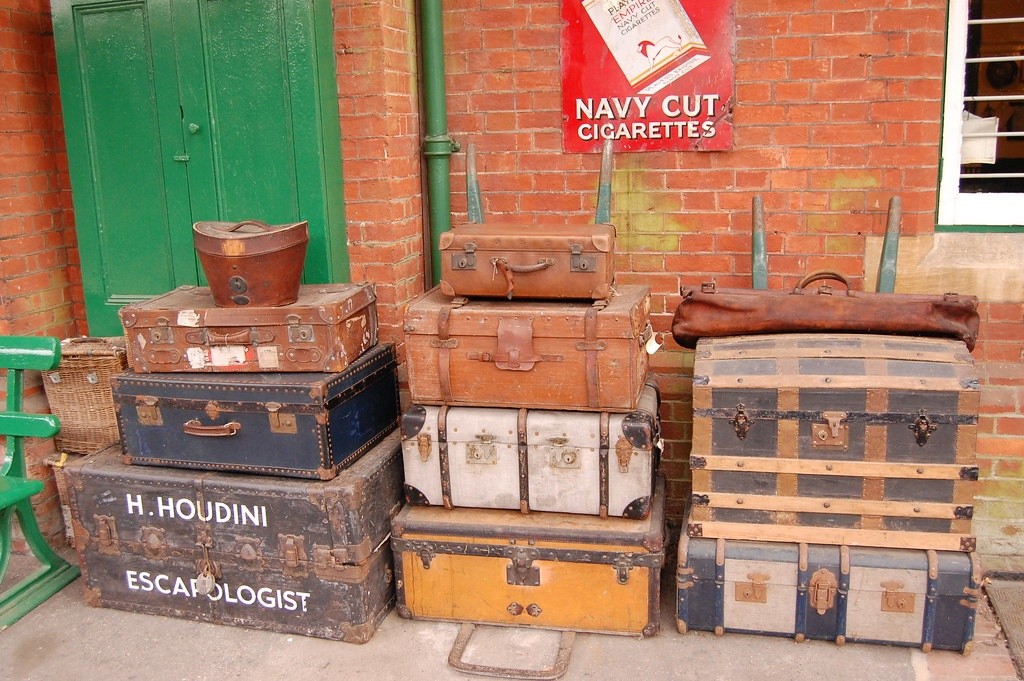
[38,333,128,456]
[41,453,126,550]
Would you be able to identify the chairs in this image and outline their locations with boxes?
[0,335,82,632]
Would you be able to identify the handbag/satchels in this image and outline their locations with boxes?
[671,268,981,353]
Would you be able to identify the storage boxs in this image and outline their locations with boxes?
[64,424,407,645]
[110,342,401,481]
[40,336,129,455]
[121,283,377,374]
[401,373,664,519]
[117,306,135,368]
[675,505,981,655]
[687,333,981,552]
[669,268,981,354]
[43,450,86,549]
[439,221,617,299]
[390,473,671,636]
[404,283,651,413]
[193,221,310,307]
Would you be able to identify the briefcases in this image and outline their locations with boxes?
[109,341,403,481]
[123,280,381,373]
[439,221,618,301]
[675,519,980,656]
[391,282,668,640]
[193,220,310,308]
